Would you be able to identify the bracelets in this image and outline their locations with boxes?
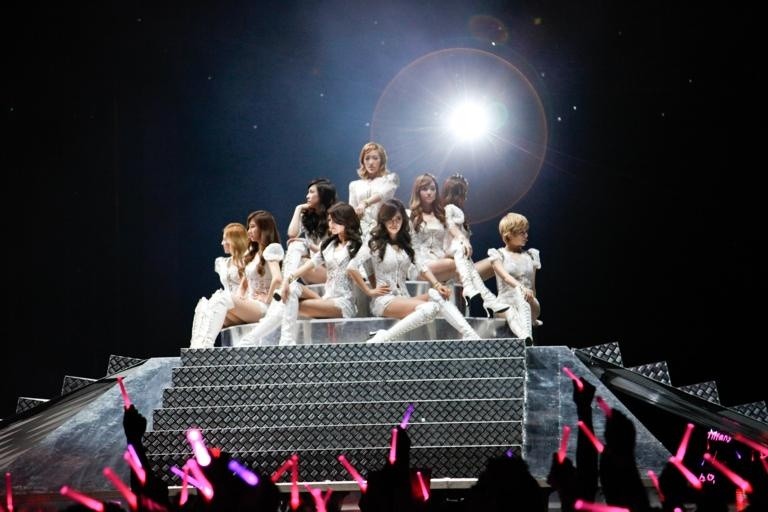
[429,280,442,288]
[518,284,525,291]
[287,275,294,283]
[301,237,310,247]
[362,198,372,206]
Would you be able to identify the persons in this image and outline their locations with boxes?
[189,210,285,347]
[409,172,510,317]
[212,222,254,290]
[487,213,543,344]
[458,233,465,242]
[440,171,496,280]
[349,142,401,239]
[237,201,362,345]
[282,178,338,286]
[349,198,482,343]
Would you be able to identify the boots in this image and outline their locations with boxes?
[368,303,439,343]
[428,289,480,341]
[280,283,302,346]
[506,286,533,346]
[280,240,307,286]
[237,298,284,347]
[451,244,508,317]
[189,288,235,349]
